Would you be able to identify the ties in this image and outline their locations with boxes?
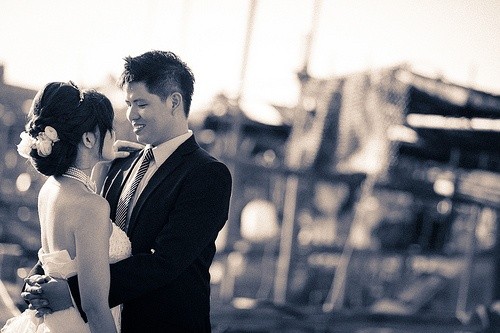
[108,147,155,239]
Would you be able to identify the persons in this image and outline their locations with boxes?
[19,45,233,332]
[0,79,145,333]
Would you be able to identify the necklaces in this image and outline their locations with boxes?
[63,167,98,191]
